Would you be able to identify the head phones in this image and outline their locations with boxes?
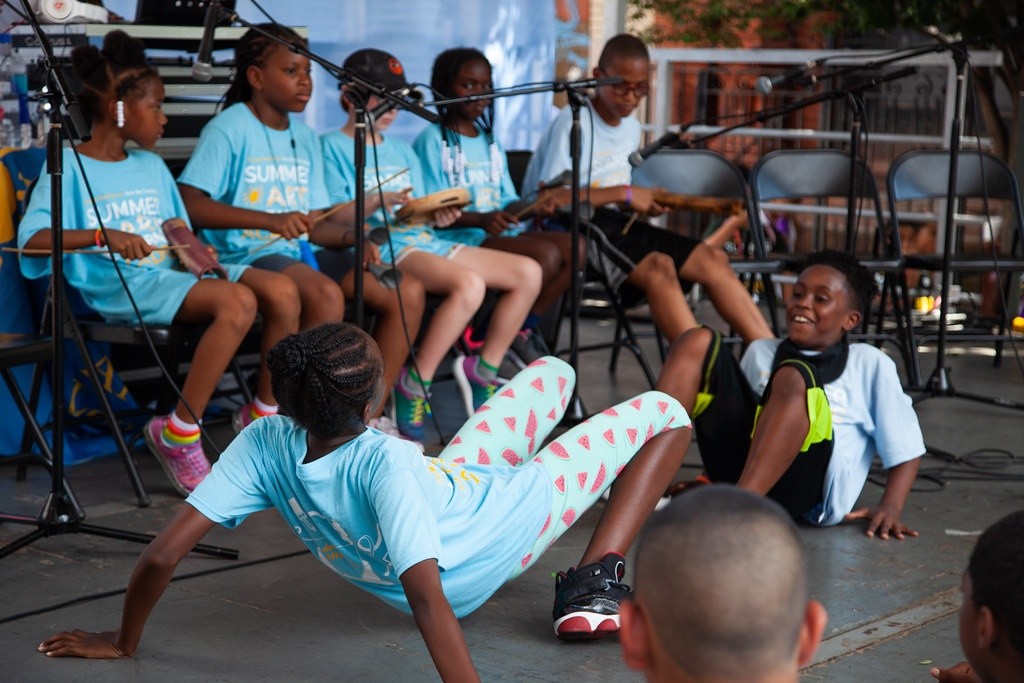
[30,1,109,26]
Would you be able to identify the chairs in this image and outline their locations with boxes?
[17,148,1024,507]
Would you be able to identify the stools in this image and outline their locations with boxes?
[0,332,84,522]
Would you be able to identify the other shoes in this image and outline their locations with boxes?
[601,480,672,510]
[231,403,261,440]
[367,415,425,455]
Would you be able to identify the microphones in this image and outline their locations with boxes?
[922,25,966,54]
[629,124,689,165]
[364,84,418,130]
[755,58,828,95]
[192,0,220,83]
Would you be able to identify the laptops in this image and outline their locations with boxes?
[101,0,237,26]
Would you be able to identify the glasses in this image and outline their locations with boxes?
[598,65,649,98]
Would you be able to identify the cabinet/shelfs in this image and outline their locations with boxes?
[650,44,1004,299]
[11,24,310,155]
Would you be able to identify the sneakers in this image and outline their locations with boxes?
[503,328,548,370]
[142,415,211,498]
[551,552,635,642]
[390,364,428,446]
[453,352,503,419]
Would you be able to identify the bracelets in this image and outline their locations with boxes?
[96,228,105,247]
[622,186,632,205]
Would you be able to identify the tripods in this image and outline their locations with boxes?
[795,33,1024,411]
[0,0,239,561]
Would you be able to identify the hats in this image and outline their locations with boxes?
[340,48,422,100]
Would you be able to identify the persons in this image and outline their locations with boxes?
[522,34,774,341]
[175,23,585,453]
[18,29,300,499]
[602,250,927,540]
[931,509,1024,683]
[619,485,826,683]
[38,323,692,683]
